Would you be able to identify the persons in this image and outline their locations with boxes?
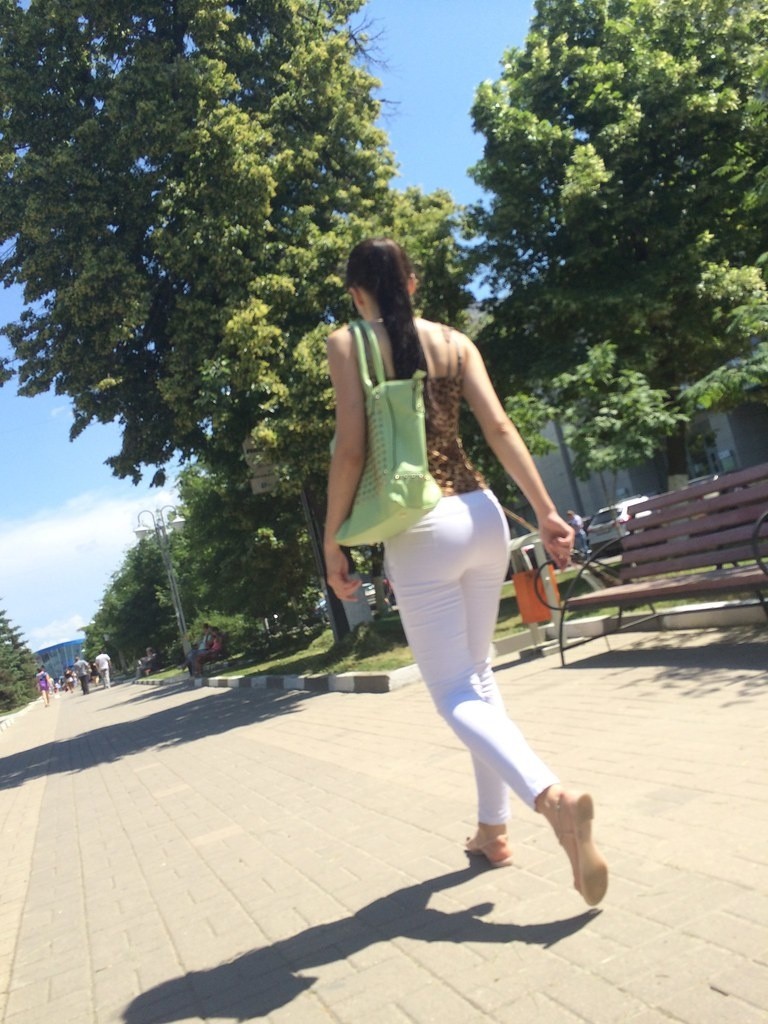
[321,236,612,907]
[35,648,113,708]
[564,508,591,560]
[136,647,155,679]
[191,623,224,679]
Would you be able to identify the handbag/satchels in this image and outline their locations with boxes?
[329,322,442,548]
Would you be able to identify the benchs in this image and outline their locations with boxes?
[534,463,768,668]
[205,632,237,670]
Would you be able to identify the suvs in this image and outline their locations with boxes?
[586,493,653,556]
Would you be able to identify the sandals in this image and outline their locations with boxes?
[464,831,515,868]
[557,790,609,906]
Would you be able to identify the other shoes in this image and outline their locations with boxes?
[177,664,186,670]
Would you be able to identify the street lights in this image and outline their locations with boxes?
[131,504,194,660]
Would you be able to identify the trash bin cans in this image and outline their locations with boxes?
[510,531,584,657]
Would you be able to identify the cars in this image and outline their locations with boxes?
[318,581,378,622]
[684,470,751,519]
[505,527,547,581]
